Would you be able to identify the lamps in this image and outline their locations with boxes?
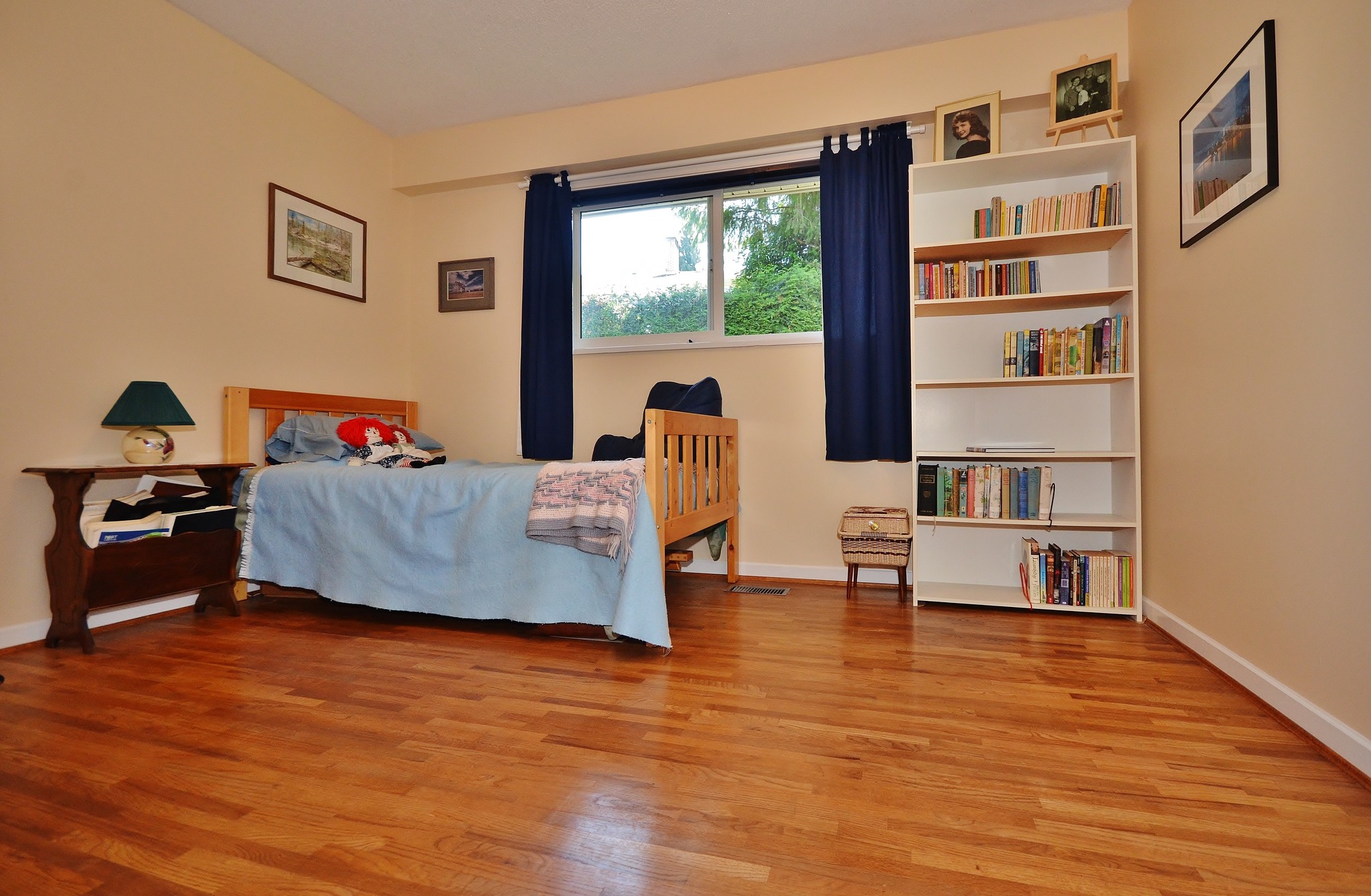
[101,380,195,465]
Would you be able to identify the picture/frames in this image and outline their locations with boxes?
[1179,18,1279,248]
[268,181,367,302]
[1046,53,1125,147]
[935,90,1002,164]
[438,257,496,313]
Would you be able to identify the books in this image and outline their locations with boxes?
[1021,536,1134,609]
[1003,312,1128,378]
[974,179,1122,239]
[966,446,1056,453]
[916,462,1053,520]
[78,474,239,550]
[914,258,1041,300]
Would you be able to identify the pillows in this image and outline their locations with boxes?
[265,412,446,462]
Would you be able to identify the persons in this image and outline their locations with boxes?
[1077,66,1098,115]
[1092,73,1110,113]
[951,109,991,160]
[1075,84,1089,117]
[1063,76,1090,121]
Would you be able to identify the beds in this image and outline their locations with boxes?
[225,383,741,652]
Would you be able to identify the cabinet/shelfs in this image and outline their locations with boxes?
[907,133,1145,625]
[22,462,258,656]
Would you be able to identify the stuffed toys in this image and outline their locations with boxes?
[336,416,439,469]
[386,423,446,465]
